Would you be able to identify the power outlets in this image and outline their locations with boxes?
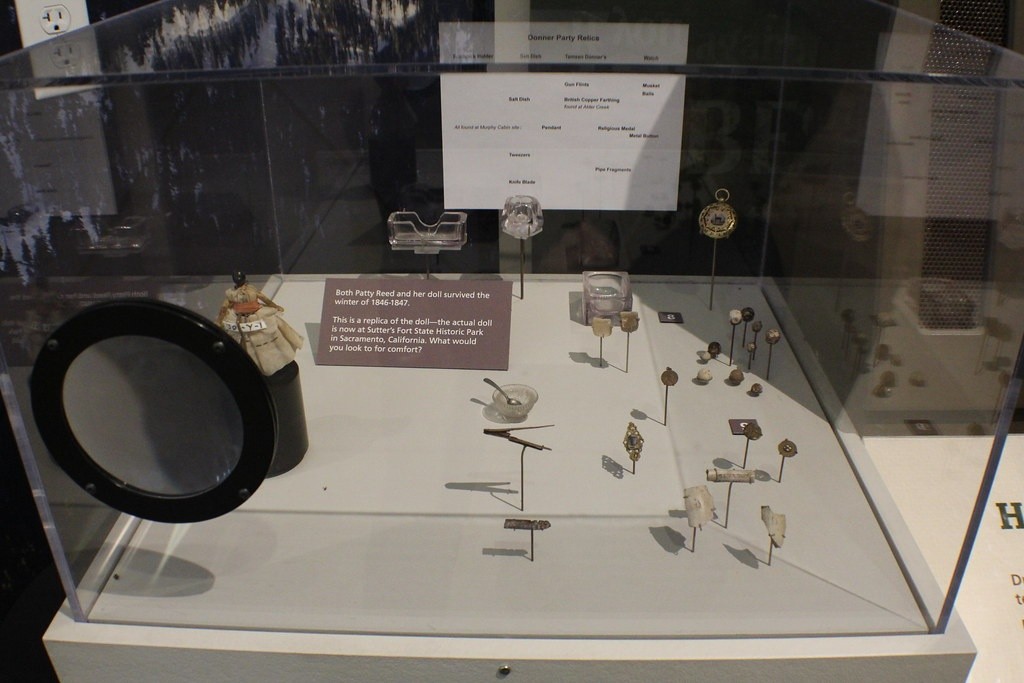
[9,0,104,101]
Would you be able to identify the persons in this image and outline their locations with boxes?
[215,269,306,377]
